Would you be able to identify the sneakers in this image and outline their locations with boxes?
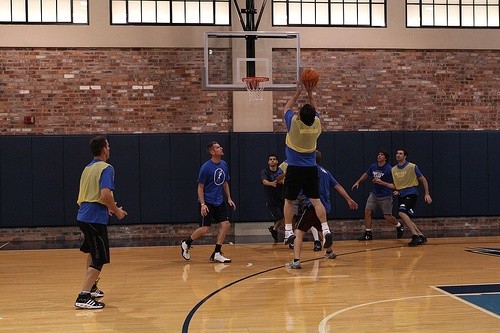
[180,239,191,260]
[395,221,404,238]
[90,284,104,297]
[356,230,373,241]
[74,293,105,310]
[209,252,232,263]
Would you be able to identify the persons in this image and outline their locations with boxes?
[372,149,433,246]
[284,85,334,269]
[75,135,127,308]
[352,151,404,240]
[181,141,236,263]
[260,150,358,258]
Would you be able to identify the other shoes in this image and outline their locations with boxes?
[268,226,278,240]
[407,234,427,246]
[323,250,337,259]
[322,229,333,248]
[283,231,296,245]
[288,240,294,248]
[285,259,301,269]
[313,240,322,251]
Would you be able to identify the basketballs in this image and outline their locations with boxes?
[301,68,319,88]
